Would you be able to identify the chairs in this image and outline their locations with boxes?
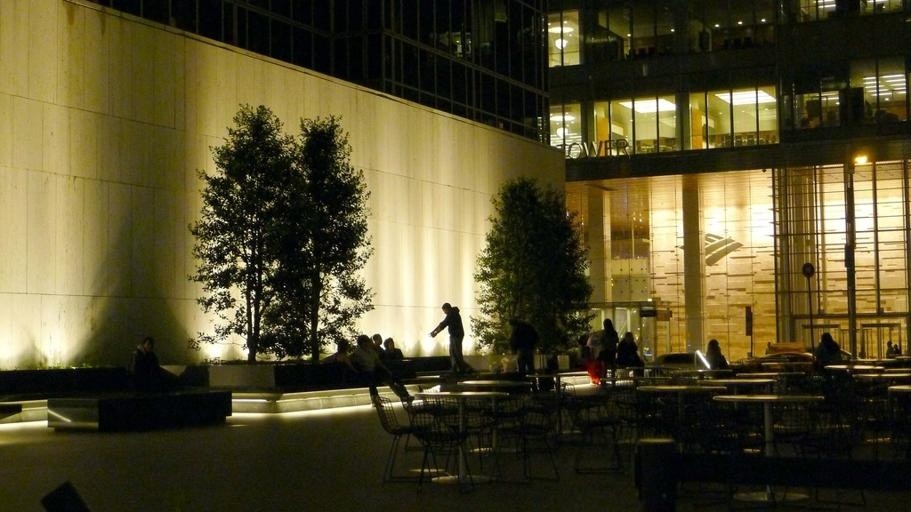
[369,361,449,500]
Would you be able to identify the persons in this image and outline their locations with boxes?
[131,334,164,392]
[509,317,538,374]
[333,331,404,361]
[784,104,901,132]
[618,331,645,377]
[885,341,902,359]
[430,302,465,372]
[707,339,728,369]
[597,317,617,385]
[815,332,842,364]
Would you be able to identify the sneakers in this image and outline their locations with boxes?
[371,401,384,407]
[402,394,415,401]
